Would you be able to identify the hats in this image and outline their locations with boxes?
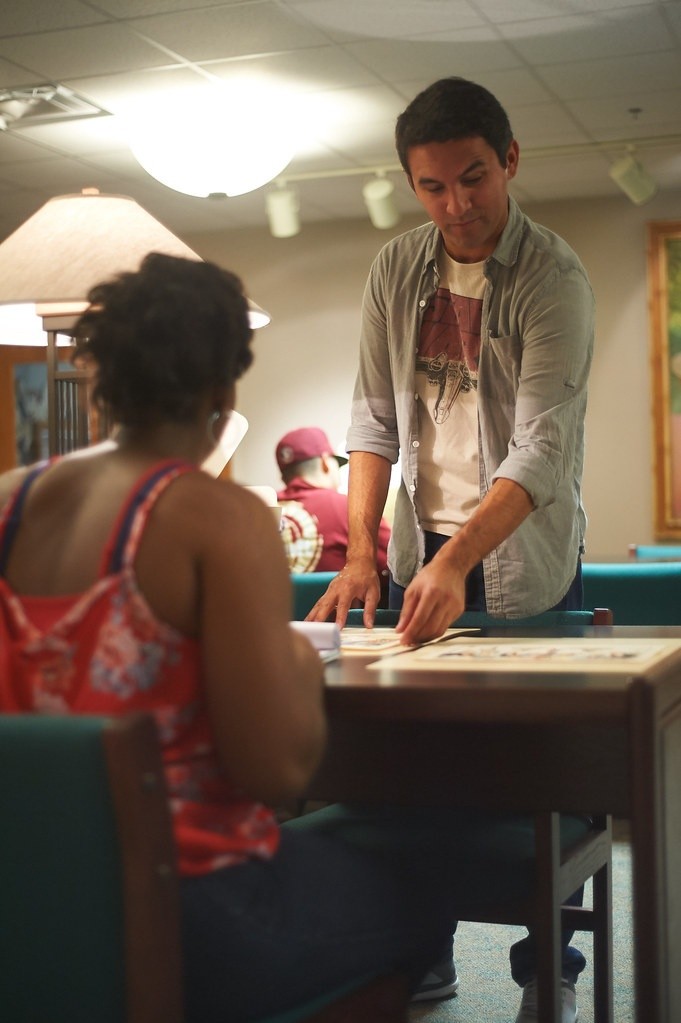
[277,426,349,469]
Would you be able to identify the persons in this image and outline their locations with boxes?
[1,252,328,874]
[305,75,597,1023]
[275,425,391,573]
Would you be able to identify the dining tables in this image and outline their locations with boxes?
[319,620,680,1023]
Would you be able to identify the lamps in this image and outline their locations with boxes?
[1,189,274,461]
[123,70,303,200]
[264,190,301,238]
[611,157,661,206]
[364,180,401,231]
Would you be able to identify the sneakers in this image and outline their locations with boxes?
[516,977,577,1022]
[412,958,459,1002]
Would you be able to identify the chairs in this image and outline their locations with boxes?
[0,709,401,1022]
[294,542,681,618]
